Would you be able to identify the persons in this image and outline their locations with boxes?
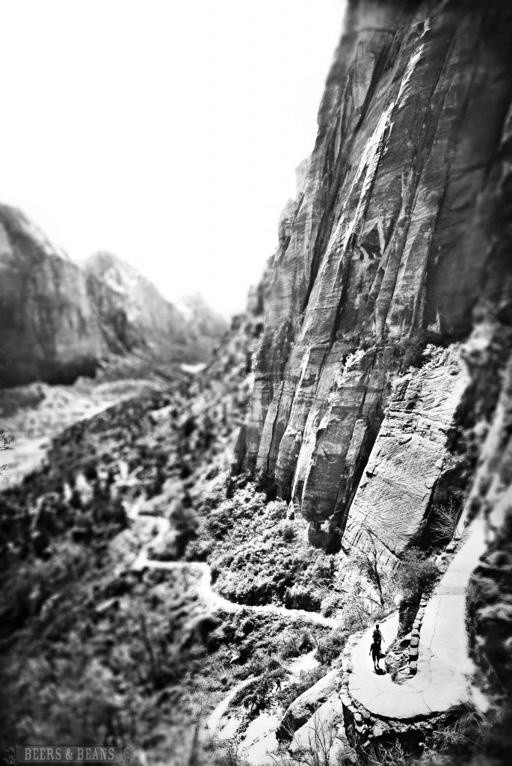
[369,638,382,670]
[372,623,382,651]
